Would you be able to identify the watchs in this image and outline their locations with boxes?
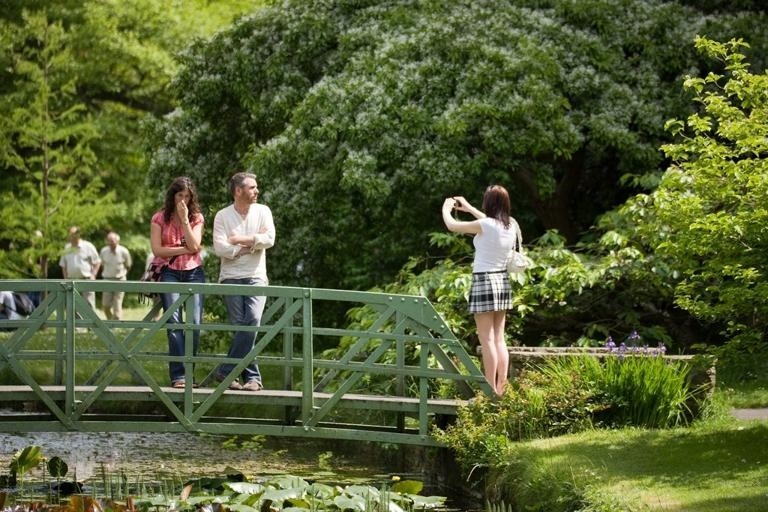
[182,219,189,226]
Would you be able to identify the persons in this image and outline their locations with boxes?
[441,184,517,396]
[149,176,207,391]
[56,224,103,334]
[212,172,277,393]
[22,229,44,309]
[99,231,133,321]
[1,290,29,332]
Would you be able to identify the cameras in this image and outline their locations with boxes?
[453,199,460,208]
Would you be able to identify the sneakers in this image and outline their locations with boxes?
[241,380,260,391]
[215,373,243,390]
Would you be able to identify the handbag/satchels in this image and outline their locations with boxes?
[506,250,527,272]
[141,264,161,298]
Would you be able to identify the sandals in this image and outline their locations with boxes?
[172,381,199,388]
